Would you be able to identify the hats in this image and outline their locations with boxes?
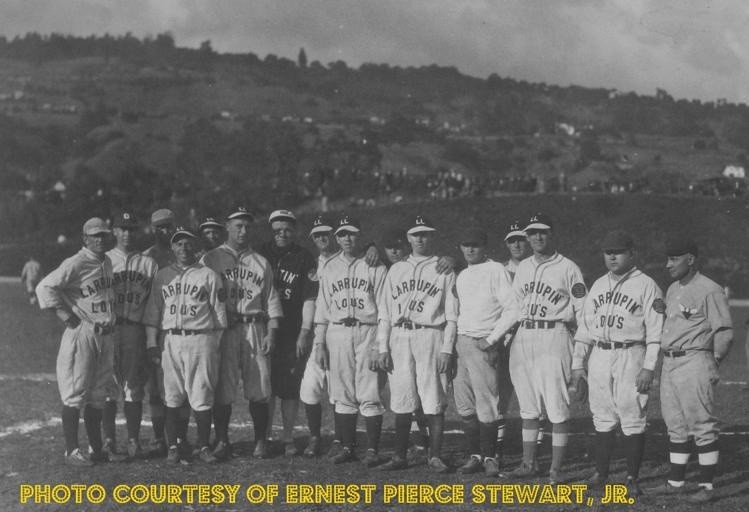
[663,235,699,256]
[268,210,297,224]
[460,227,487,247]
[383,215,436,244]
[83,205,254,244]
[600,228,634,251]
[308,213,361,237]
[505,212,554,240]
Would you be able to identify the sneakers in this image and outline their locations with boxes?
[585,472,608,488]
[99,436,286,465]
[684,487,715,506]
[619,479,640,502]
[63,448,95,468]
[643,483,690,501]
[303,437,449,473]
[451,453,569,484]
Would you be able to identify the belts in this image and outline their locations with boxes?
[663,349,686,358]
[231,316,260,322]
[167,328,201,335]
[592,341,635,349]
[397,322,423,330]
[334,321,368,327]
[95,325,115,335]
[520,319,556,329]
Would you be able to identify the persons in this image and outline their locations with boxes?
[313,213,389,466]
[450,230,517,478]
[375,212,459,473]
[654,241,734,504]
[497,210,589,486]
[495,220,545,460]
[20,255,41,306]
[35,205,381,467]
[363,227,459,452]
[568,229,663,500]
